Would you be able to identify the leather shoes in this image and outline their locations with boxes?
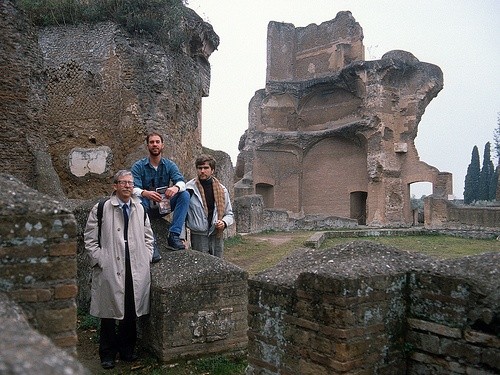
[120,353,139,364]
[102,360,114,368]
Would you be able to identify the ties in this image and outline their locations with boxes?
[122,204,128,250]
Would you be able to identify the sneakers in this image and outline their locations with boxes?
[152,244,162,262]
[164,231,185,250]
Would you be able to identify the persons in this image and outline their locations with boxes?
[130,133,190,264]
[185,155,234,258]
[84,169,155,369]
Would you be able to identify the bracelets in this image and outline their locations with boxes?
[175,186,180,192]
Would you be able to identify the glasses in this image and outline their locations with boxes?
[117,181,134,186]
